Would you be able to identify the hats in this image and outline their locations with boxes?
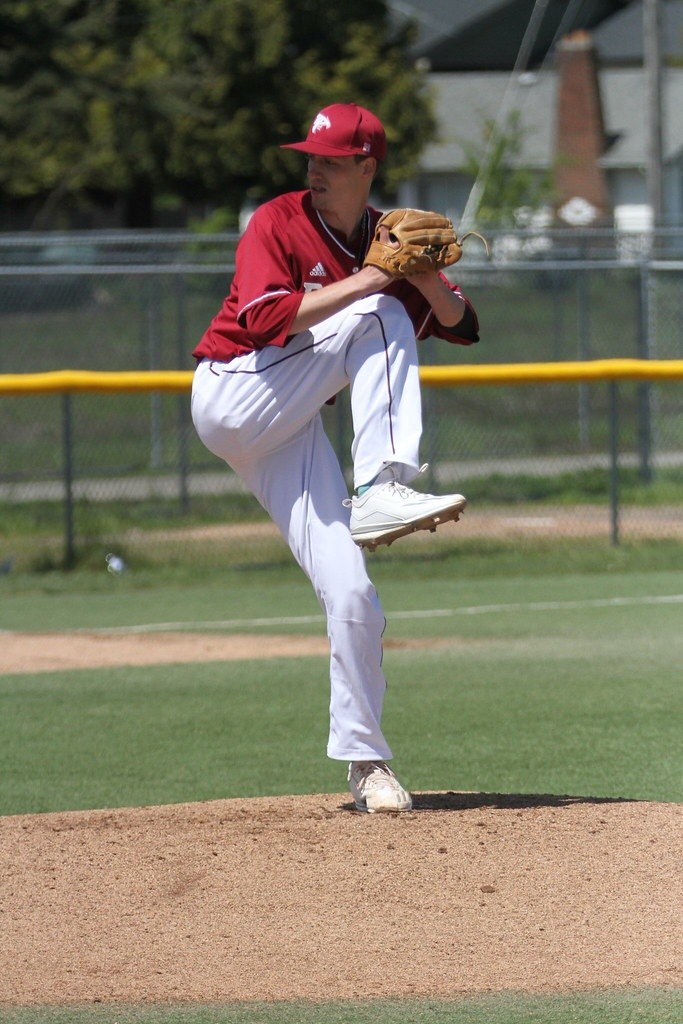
[280,102,386,161]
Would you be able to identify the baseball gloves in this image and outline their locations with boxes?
[361,207,463,282]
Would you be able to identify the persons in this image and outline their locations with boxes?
[189,103,480,813]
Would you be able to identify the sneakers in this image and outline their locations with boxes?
[347,761,412,813]
[341,463,467,550]
